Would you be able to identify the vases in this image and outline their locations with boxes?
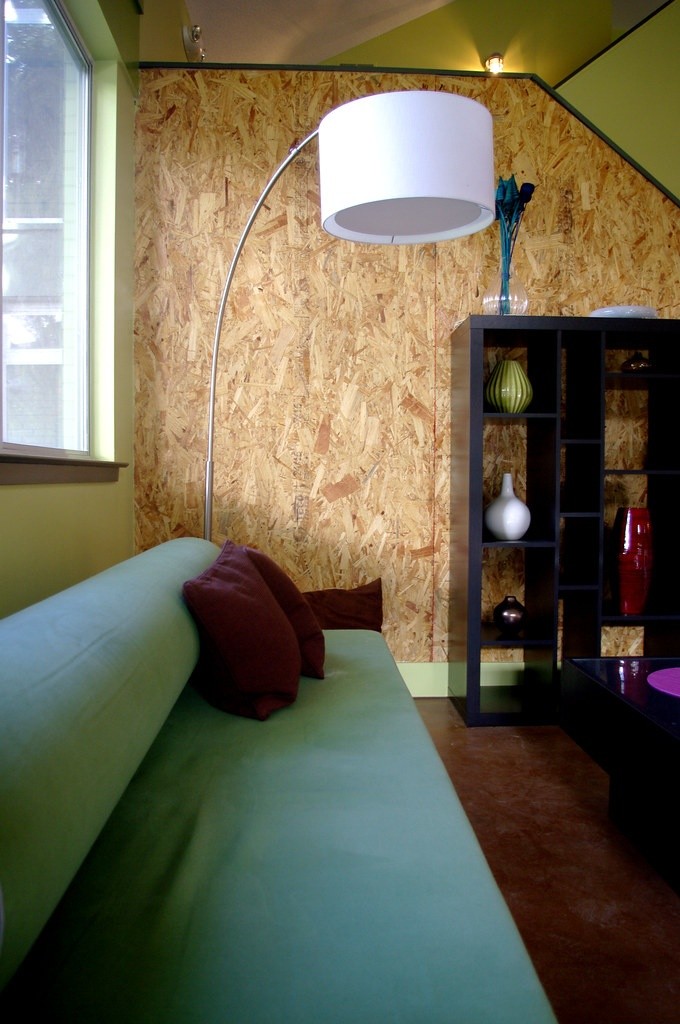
[479,269,530,317]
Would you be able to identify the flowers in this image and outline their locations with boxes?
[485,178,538,268]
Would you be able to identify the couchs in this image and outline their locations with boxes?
[0,537,559,1022]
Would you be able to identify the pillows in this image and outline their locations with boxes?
[242,545,326,680]
[304,578,385,634]
[182,540,302,719]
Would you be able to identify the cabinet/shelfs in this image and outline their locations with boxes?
[450,314,680,728]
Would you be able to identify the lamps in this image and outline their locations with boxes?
[182,23,206,65]
[201,89,494,539]
[484,52,506,79]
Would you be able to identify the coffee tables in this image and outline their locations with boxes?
[563,657,680,894]
